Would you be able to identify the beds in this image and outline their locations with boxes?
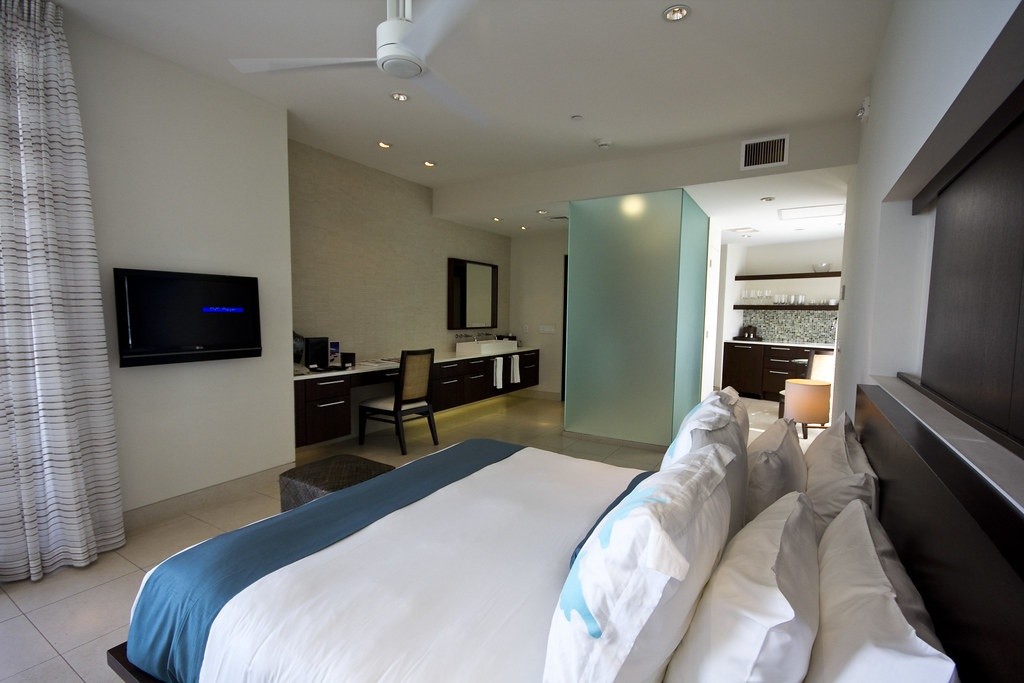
[106,369,1024,683]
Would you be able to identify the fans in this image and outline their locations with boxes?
[229,0,479,114]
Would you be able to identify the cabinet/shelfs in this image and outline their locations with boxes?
[722,342,815,401]
[294,349,539,447]
[733,271,841,311]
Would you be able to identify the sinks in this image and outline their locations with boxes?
[456,340,517,355]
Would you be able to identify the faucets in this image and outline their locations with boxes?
[472,336,478,344]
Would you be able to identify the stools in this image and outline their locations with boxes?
[279,453,395,511]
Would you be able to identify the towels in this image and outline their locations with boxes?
[493,357,503,389]
[510,355,520,383]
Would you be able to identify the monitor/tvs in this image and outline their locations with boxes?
[113,268,262,368]
[304,336,330,372]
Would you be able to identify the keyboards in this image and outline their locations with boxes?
[329,366,348,370]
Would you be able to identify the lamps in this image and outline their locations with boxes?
[785,378,831,439]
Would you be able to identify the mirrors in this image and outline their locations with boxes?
[447,258,498,329]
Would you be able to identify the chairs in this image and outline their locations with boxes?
[359,348,439,455]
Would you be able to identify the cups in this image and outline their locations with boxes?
[741,289,771,304]
[772,294,836,305]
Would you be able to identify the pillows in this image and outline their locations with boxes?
[745,417,804,526]
[806,412,876,539]
[808,498,960,683]
[661,491,822,683]
[662,385,752,541]
[543,444,737,683]
